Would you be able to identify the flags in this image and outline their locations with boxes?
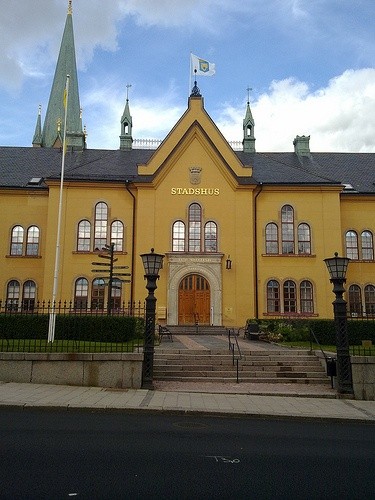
[191,53,216,77]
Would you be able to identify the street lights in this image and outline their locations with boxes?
[322,251,358,400]
[139,248,167,392]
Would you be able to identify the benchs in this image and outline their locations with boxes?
[158,325,174,343]
[244,323,270,342]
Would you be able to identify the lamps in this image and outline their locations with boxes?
[225,260,232,269]
